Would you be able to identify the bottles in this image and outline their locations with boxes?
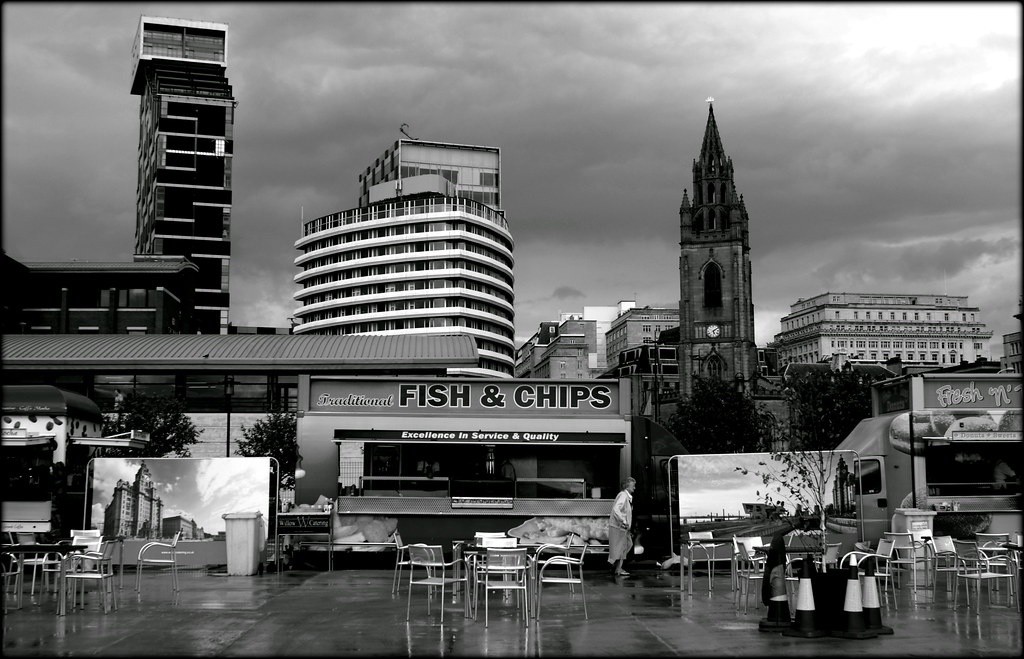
[282,499,291,512]
[1012,532,1023,548]
[350,484,356,496]
[323,497,334,512]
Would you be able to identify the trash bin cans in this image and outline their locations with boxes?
[221,512,262,576]
[894,507,937,569]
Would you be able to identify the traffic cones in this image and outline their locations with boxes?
[834,552,879,640]
[757,531,793,631]
[782,556,828,639]
[862,556,895,636]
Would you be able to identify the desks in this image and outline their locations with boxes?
[1,544,89,616]
[750,546,825,610]
[461,546,536,620]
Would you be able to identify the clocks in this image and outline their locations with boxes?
[706,324,721,339]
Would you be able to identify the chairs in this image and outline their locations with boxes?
[1,531,182,616]
[390,532,588,620]
[682,532,1022,615]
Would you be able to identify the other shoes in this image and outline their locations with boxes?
[615,568,630,575]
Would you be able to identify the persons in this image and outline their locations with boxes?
[993,455,1016,494]
[608,477,636,576]
[935,476,952,495]
[416,451,440,477]
